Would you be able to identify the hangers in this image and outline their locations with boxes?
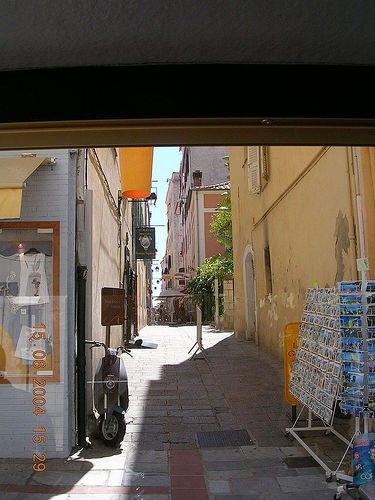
[15,247,50,259]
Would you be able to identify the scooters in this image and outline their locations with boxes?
[81,338,142,449]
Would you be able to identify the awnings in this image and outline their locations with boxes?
[0,156,56,219]
